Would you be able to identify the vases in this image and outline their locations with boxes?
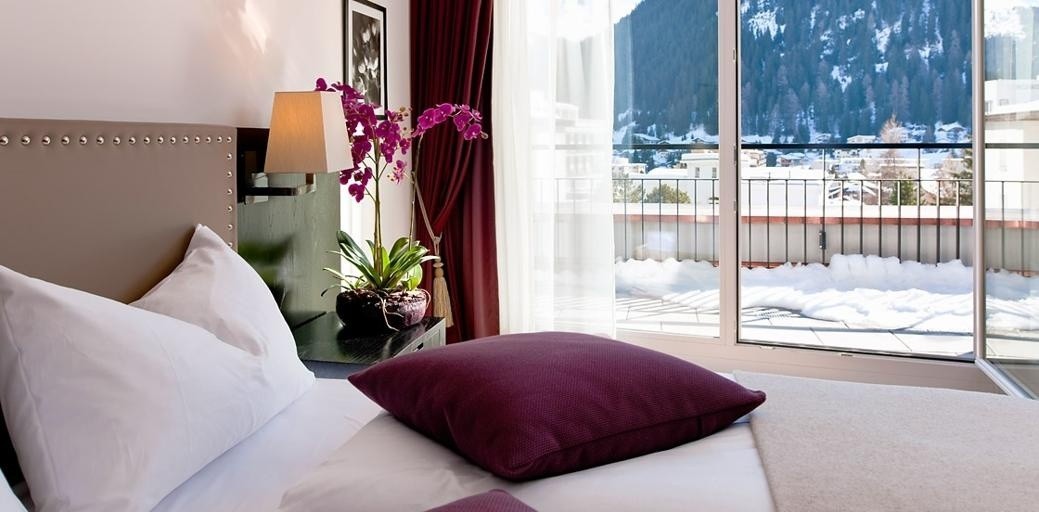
[336,291,428,327]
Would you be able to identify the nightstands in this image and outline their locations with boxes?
[298,312,446,367]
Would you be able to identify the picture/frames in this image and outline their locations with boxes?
[343,0,388,120]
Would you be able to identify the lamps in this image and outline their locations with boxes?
[242,90,354,197]
[315,76,488,299]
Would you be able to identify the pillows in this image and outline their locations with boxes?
[0,226,315,512]
[422,488,538,512]
[348,330,769,483]
[278,411,496,512]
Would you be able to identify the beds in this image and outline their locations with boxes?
[0,117,1039,512]
